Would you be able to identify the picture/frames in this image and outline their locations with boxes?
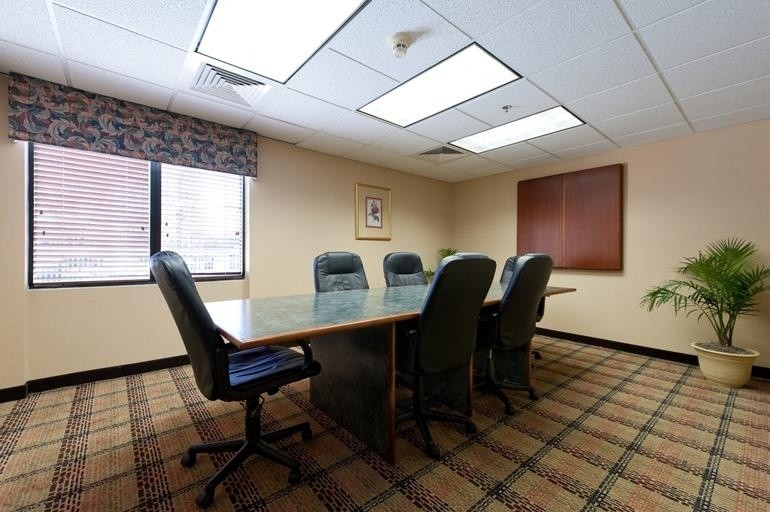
[354,182,393,241]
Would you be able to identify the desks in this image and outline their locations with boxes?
[203,282,577,466]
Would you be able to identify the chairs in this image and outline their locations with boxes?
[383,252,429,287]
[474,252,555,416]
[313,251,371,292]
[485,256,546,362]
[151,251,322,508]
[392,251,497,461]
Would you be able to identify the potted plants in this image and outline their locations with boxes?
[638,238,770,389]
